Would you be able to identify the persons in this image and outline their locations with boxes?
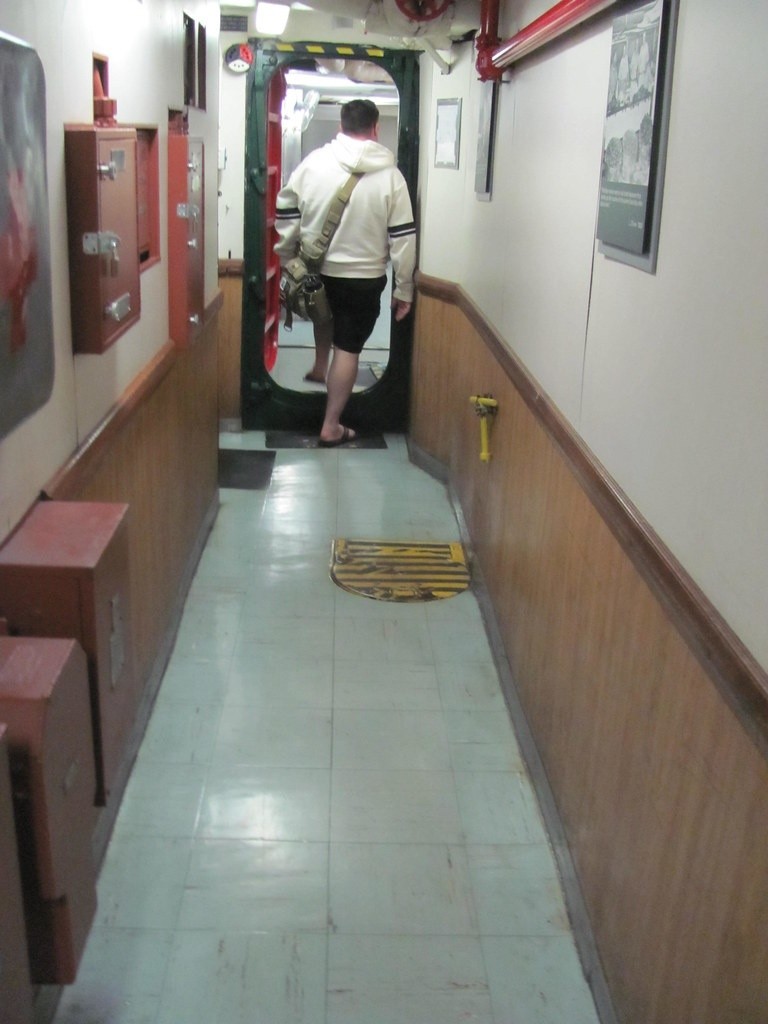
[272,100,417,446]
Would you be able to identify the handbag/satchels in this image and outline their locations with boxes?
[277,255,332,332]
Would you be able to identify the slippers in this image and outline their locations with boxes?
[304,373,326,384]
[318,424,358,447]
